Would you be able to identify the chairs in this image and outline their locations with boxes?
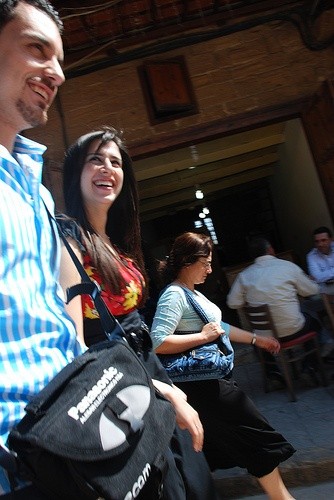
[241,291,334,401]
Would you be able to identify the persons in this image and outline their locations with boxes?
[0,0,214,500]
[226,236,322,389]
[150,232,296,500]
[305,226,334,358]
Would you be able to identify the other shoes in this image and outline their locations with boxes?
[270,372,287,391]
[300,368,319,388]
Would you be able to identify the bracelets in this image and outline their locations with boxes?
[251,333,256,344]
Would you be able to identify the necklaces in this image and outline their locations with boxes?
[91,224,110,240]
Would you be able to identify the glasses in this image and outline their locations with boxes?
[197,260,212,268]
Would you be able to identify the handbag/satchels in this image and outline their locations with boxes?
[5,339,176,500]
[156,283,234,382]
